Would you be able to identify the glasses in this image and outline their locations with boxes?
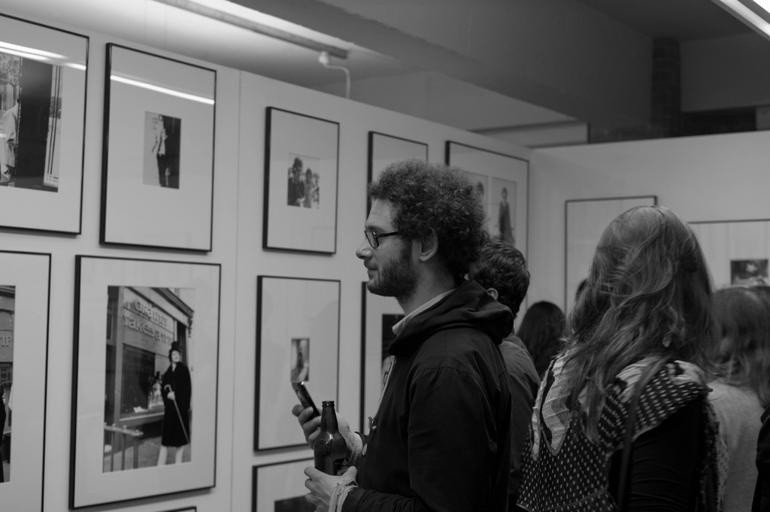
[364,228,402,249]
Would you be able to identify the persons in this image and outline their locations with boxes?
[288,158,305,206]
[498,187,513,236]
[712,287,770,512]
[515,300,566,381]
[515,204,723,509]
[574,278,587,303]
[466,237,540,511]
[155,341,191,465]
[0,100,21,184]
[751,404,769,512]
[732,258,769,287]
[296,352,303,373]
[302,168,314,208]
[293,158,515,511]
[150,115,173,187]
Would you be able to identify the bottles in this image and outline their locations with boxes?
[311,400,350,476]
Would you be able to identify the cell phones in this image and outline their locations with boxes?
[290,380,318,417]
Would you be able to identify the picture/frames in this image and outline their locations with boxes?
[686,218,769,294]
[359,280,406,438]
[564,194,657,318]
[445,140,530,264]
[0,13,90,235]
[367,129,429,221]
[262,106,340,254]
[98,42,217,254]
[0,250,52,512]
[254,275,341,471]
[69,254,222,512]
[252,454,315,512]
